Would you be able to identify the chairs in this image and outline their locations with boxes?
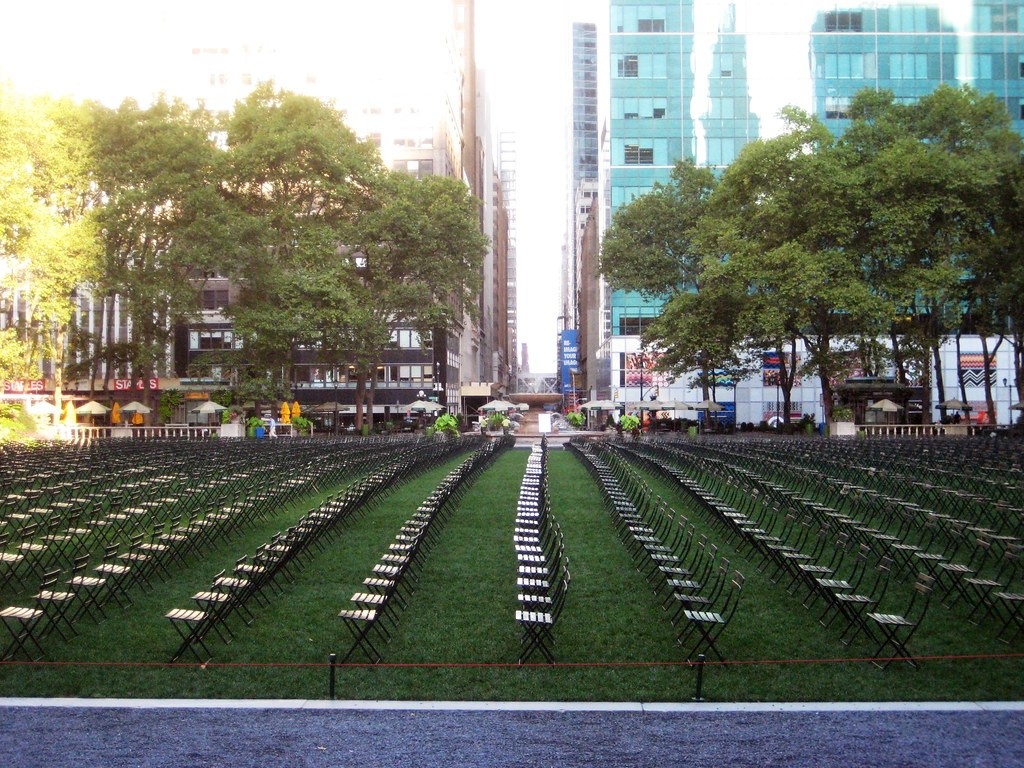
[0,433,1024,674]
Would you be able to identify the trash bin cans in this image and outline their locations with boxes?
[362,424,369,437]
[256,427,264,438]
[819,422,830,436]
[246,427,256,438]
[688,427,698,438]
[805,424,814,434]
[426,427,434,437]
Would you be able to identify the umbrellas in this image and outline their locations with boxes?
[578,398,727,437]
[117,401,154,423]
[27,400,63,435]
[477,399,519,411]
[869,399,903,425]
[280,402,290,424]
[74,400,112,426]
[402,399,446,433]
[113,402,121,423]
[310,401,350,434]
[189,402,228,436]
[292,401,300,417]
[935,398,972,424]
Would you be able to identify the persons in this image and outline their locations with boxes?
[269,416,278,440]
[952,411,962,424]
[478,417,488,435]
[500,416,512,435]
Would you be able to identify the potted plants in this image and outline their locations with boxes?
[486,414,504,436]
[800,412,815,434]
[619,414,640,441]
[686,420,697,435]
[829,408,856,435]
[246,417,263,438]
[221,407,248,437]
